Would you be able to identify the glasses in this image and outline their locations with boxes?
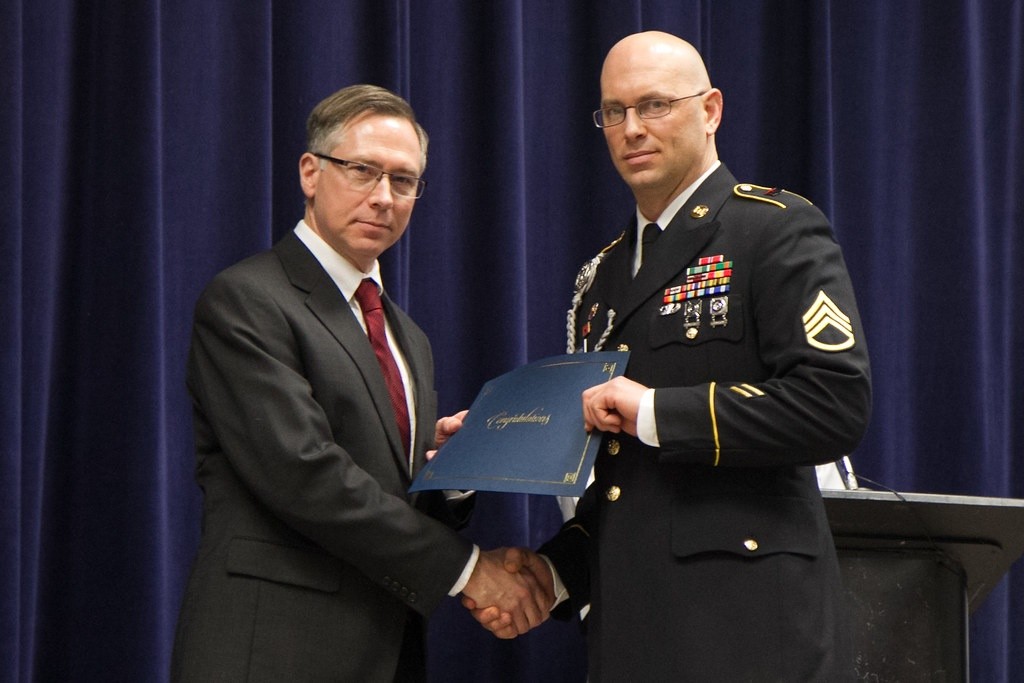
[312,152,425,199]
[593,93,705,128]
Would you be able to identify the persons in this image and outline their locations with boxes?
[459,30,872,683]
[168,86,552,683]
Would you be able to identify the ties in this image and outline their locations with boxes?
[354,278,411,470]
[641,223,662,266]
